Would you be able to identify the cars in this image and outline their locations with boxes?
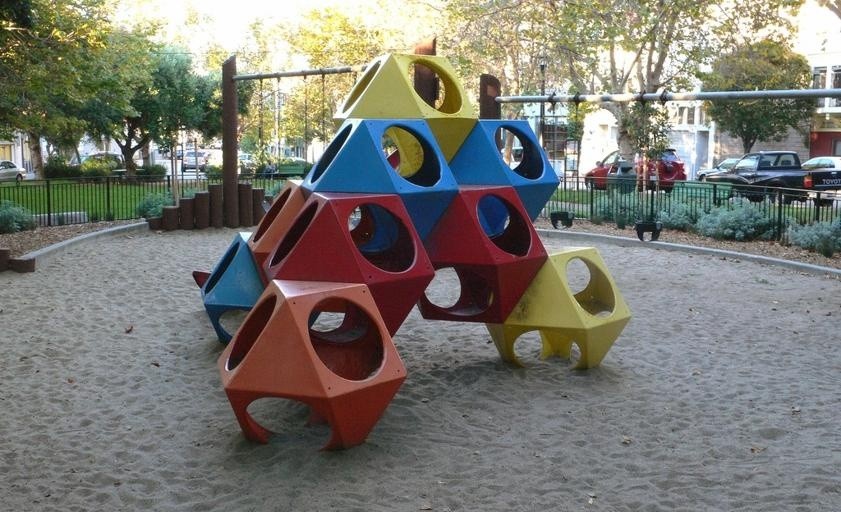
[801,156,841,170]
[703,150,841,205]
[696,157,776,183]
[0,160,27,182]
[164,143,263,180]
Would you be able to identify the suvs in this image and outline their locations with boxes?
[65,151,126,174]
[584,146,687,193]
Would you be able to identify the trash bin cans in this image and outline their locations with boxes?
[606,160,637,200]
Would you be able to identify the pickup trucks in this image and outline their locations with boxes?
[501,146,564,186]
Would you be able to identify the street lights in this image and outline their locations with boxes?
[538,59,547,150]
